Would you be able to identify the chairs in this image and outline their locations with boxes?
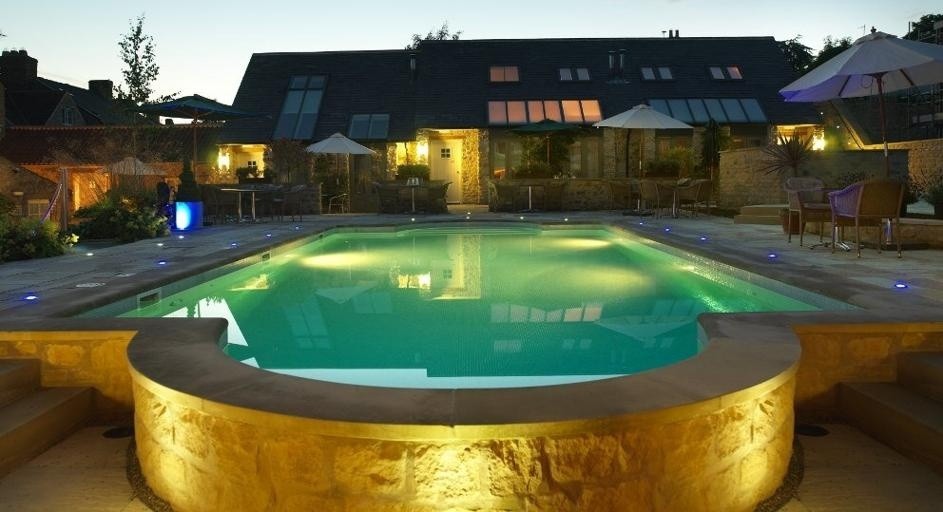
[488,181,570,215]
[200,166,349,231]
[827,177,910,258]
[371,181,451,217]
[785,176,847,248]
[605,175,715,224]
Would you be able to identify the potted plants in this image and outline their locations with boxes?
[173,147,202,233]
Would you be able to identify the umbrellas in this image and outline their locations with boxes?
[778,29,942,251]
[591,103,696,217]
[125,94,252,185]
[505,116,590,180]
[93,156,167,177]
[299,131,377,190]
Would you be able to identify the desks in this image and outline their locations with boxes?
[807,184,865,254]
